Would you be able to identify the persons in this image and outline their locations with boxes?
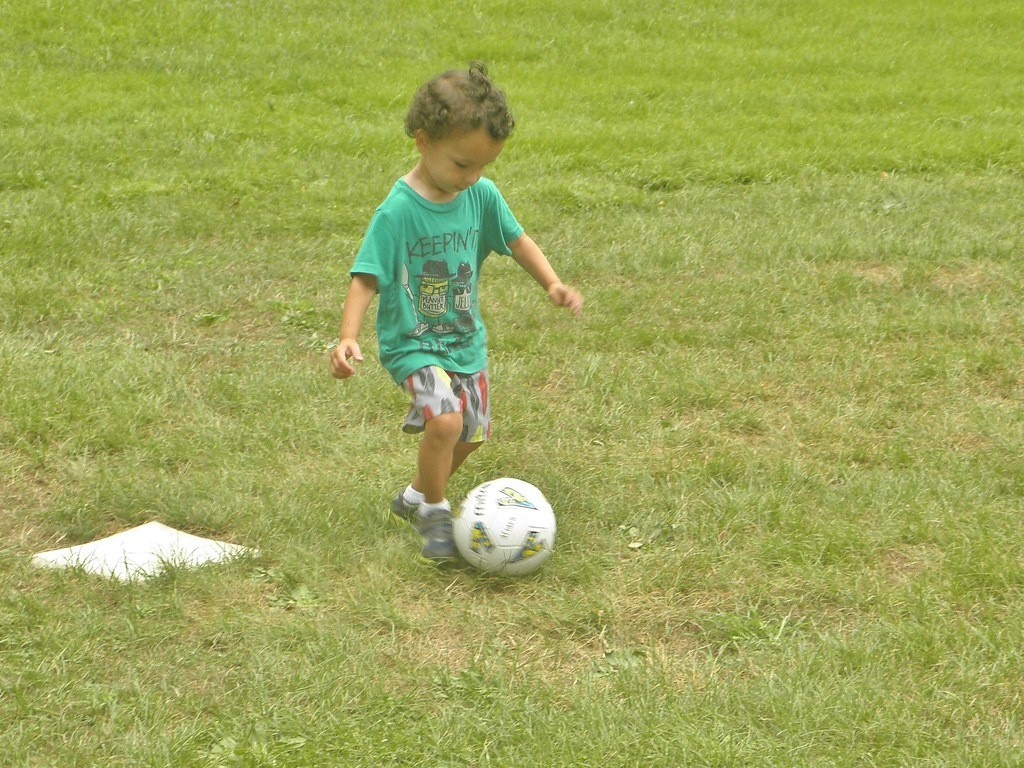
[327,59,587,566]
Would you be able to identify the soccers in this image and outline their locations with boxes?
[454,477,555,575]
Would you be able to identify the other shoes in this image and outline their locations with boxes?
[389,490,419,523]
[414,510,457,563]
